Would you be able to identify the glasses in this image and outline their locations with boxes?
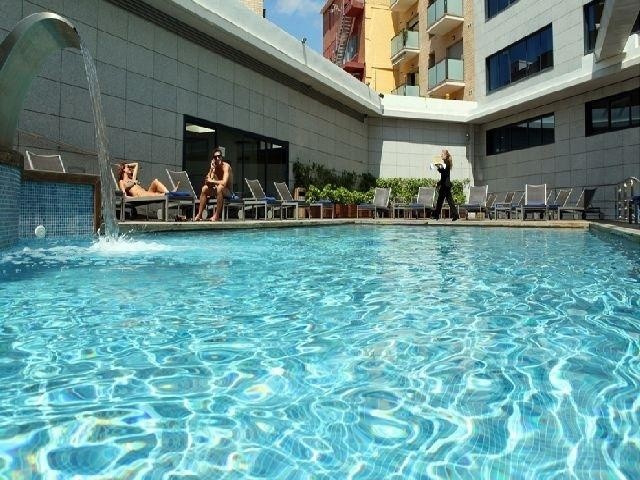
[213,155,221,159]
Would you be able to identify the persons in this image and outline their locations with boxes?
[119,162,179,198]
[191,150,234,220]
[429,149,460,222]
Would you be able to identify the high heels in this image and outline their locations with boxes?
[430,214,439,220]
[452,214,459,221]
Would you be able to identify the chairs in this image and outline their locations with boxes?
[26,151,65,173]
[615,177,640,224]
[111,165,601,222]
